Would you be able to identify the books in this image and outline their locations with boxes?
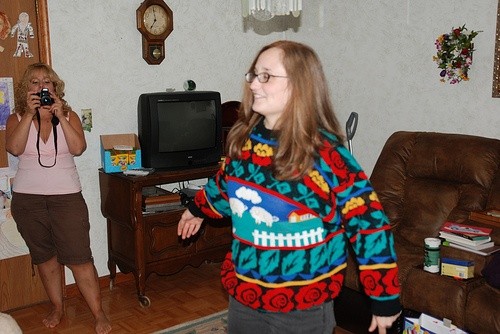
[439,221,500,256]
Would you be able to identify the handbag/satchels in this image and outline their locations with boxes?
[405,314,466,334]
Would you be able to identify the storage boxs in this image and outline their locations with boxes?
[99,131,143,174]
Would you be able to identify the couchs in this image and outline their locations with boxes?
[359,129,500,334]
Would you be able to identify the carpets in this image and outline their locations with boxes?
[155,309,228,334]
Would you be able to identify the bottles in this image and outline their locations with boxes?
[423,237,441,273]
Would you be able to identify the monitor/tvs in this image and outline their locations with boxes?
[138,90,222,171]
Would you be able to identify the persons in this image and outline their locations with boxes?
[178,41,403,334]
[5,63,112,334]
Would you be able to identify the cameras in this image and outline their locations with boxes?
[32,89,55,108]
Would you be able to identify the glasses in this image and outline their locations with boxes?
[245,72,290,82]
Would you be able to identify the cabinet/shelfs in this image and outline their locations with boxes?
[96,165,236,307]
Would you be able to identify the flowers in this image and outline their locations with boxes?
[434,22,484,83]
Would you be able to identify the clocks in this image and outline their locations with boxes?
[134,1,174,65]
